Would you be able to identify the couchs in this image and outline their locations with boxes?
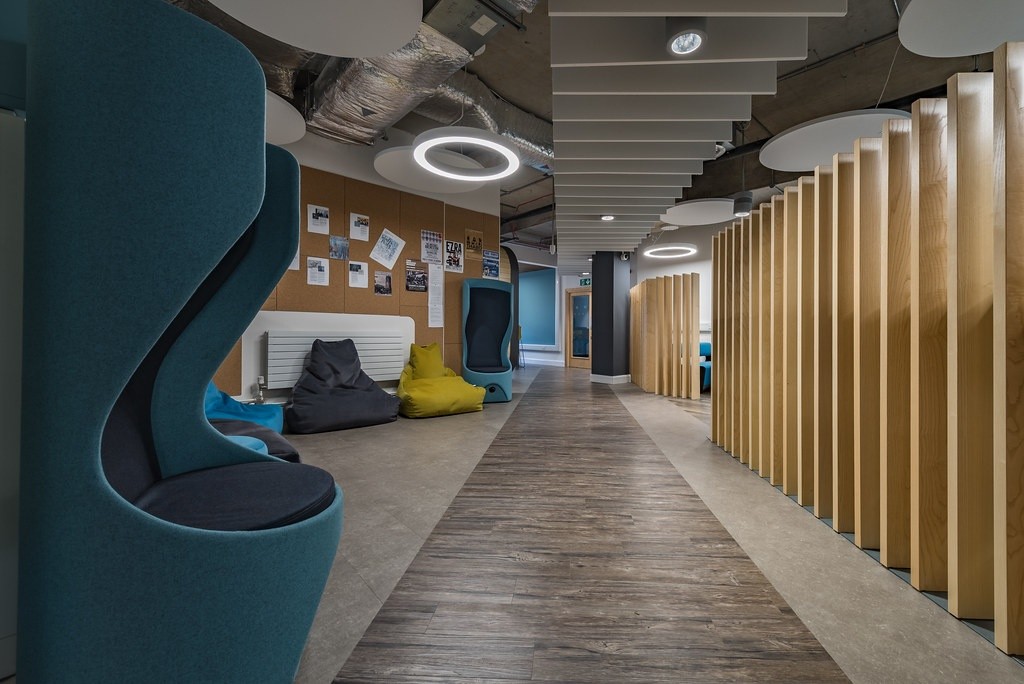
[205,380,284,438]
[397,342,487,419]
[284,339,399,435]
[204,419,304,462]
[1,0,345,683]
[461,278,513,404]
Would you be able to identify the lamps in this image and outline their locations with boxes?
[600,215,614,221]
[663,17,709,60]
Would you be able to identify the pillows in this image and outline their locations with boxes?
[411,342,444,379]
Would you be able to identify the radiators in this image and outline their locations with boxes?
[262,329,406,391]
